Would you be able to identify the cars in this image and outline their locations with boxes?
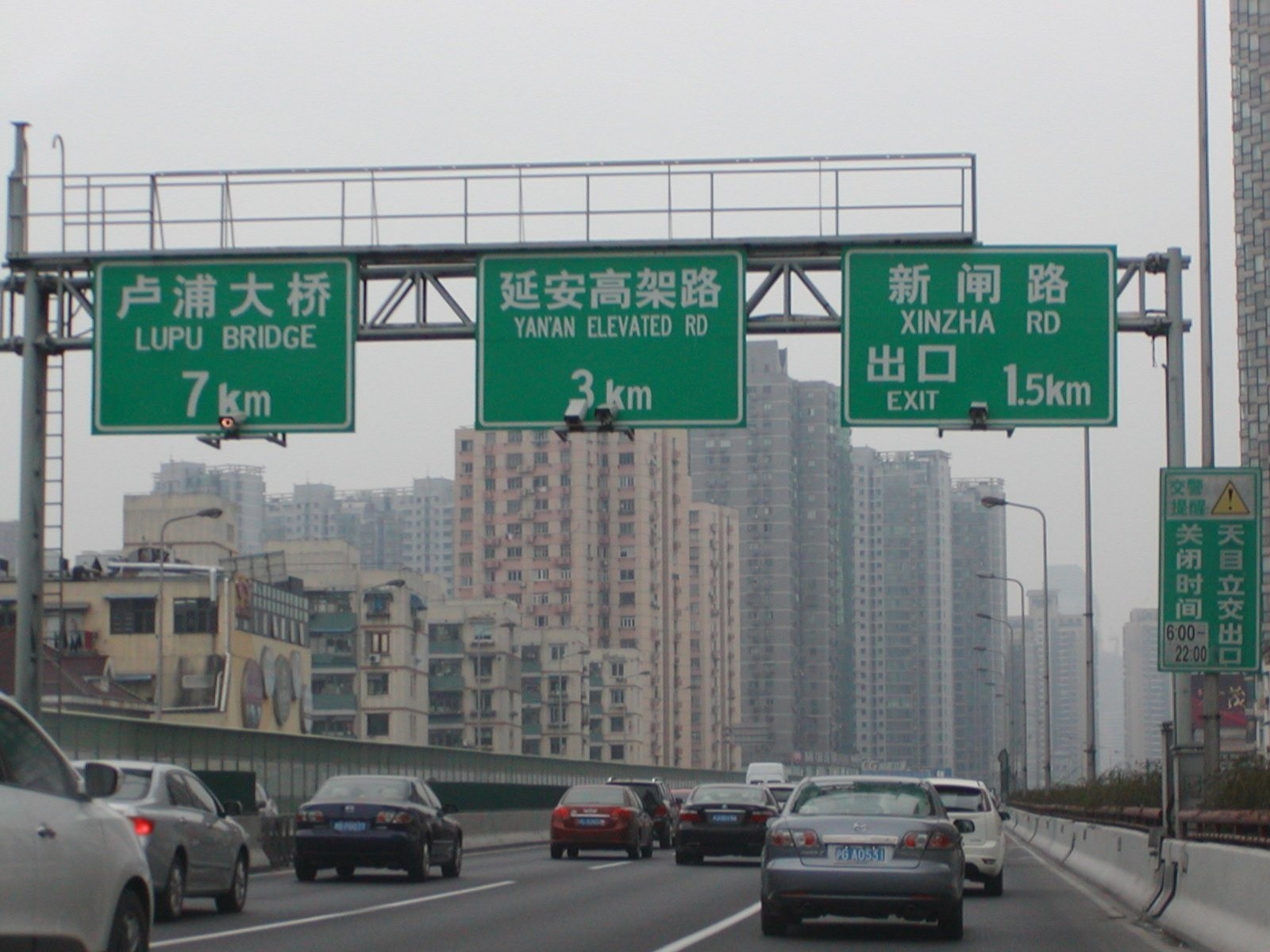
[74,760,252,921]
[549,782,656,860]
[674,784,787,866]
[669,788,693,811]
[0,689,158,952]
[254,783,281,819]
[758,775,976,941]
[744,761,798,809]
[292,774,465,882]
[921,777,1012,899]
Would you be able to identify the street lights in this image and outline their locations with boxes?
[155,506,223,717]
[980,495,1052,797]
[623,669,652,763]
[476,619,518,754]
[974,571,1031,803]
[976,610,1017,790]
[558,648,594,755]
[356,578,406,740]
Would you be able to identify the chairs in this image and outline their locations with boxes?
[706,789,721,802]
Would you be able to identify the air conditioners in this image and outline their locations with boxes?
[516,464,536,520]
[524,534,535,543]
[524,604,536,614]
[368,653,382,665]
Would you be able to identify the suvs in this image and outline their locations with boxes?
[606,776,684,850]
